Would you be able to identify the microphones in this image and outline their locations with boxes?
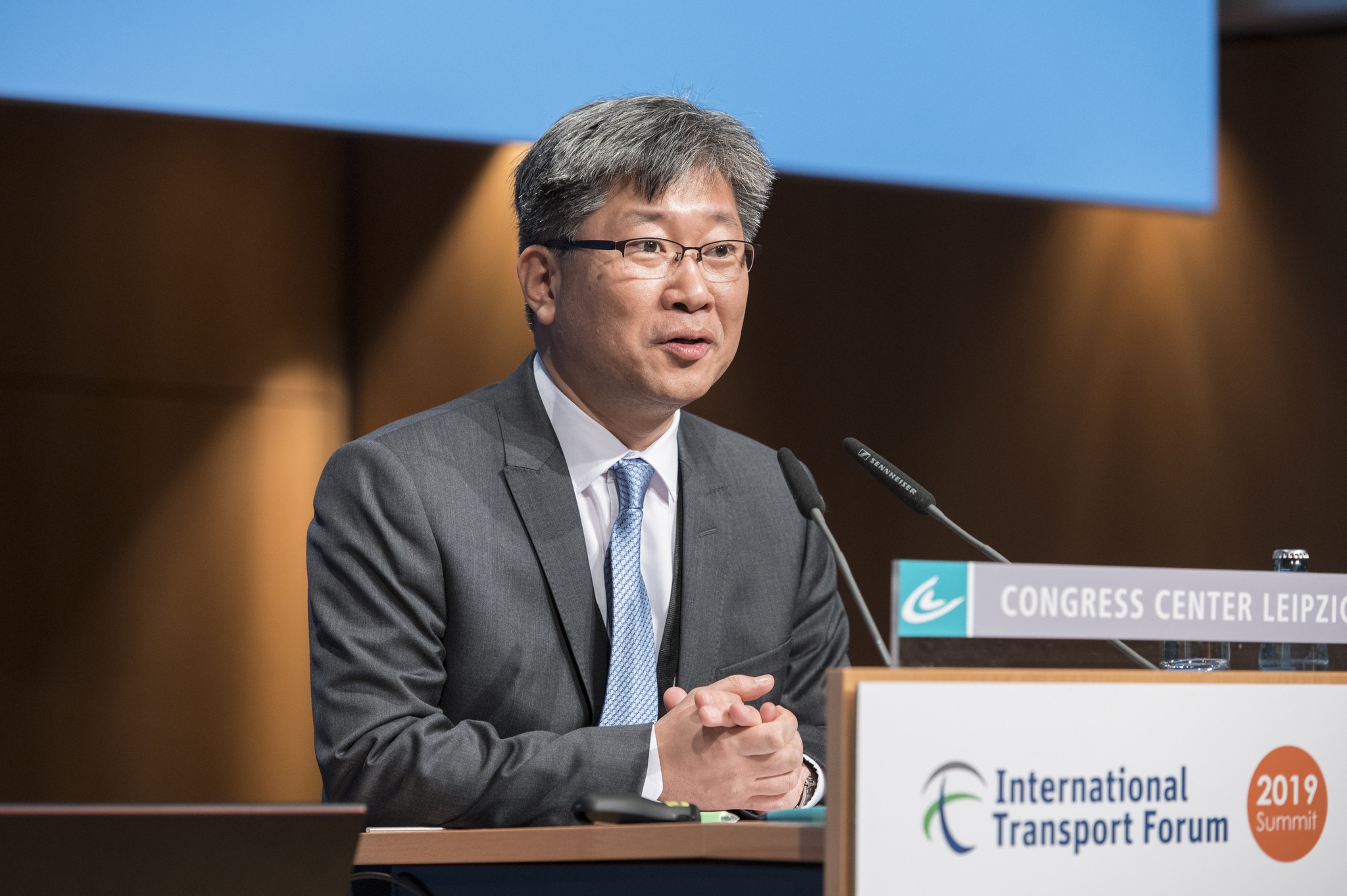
[841,435,1159,673]
[776,444,896,667]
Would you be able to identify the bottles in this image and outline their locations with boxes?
[1258,549,1329,671]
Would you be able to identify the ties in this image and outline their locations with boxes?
[597,457,659,727]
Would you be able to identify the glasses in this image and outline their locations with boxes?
[550,237,756,282]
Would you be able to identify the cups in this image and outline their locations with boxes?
[1159,640,1231,672]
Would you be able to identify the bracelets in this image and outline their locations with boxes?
[794,762,816,809]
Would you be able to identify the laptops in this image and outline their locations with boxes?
[0,798,367,896]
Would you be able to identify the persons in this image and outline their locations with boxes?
[306,94,851,830]
